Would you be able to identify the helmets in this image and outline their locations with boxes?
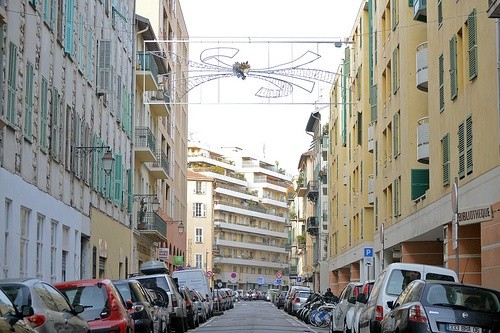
[327,288,331,291]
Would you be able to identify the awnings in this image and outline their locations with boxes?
[305,111,319,137]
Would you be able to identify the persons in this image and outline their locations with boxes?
[248,289,253,296]
[325,288,333,297]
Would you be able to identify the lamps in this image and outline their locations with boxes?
[134,193,160,213]
[71,146,115,176]
[166,220,184,235]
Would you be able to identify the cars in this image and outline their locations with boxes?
[211,280,403,333]
[128,260,212,333]
[380,279,500,333]
[110,278,163,333]
[0,285,40,333]
[49,278,136,333]
[0,277,91,333]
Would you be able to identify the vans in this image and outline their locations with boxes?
[171,268,214,318]
[357,262,462,333]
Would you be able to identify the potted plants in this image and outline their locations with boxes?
[137,198,148,230]
